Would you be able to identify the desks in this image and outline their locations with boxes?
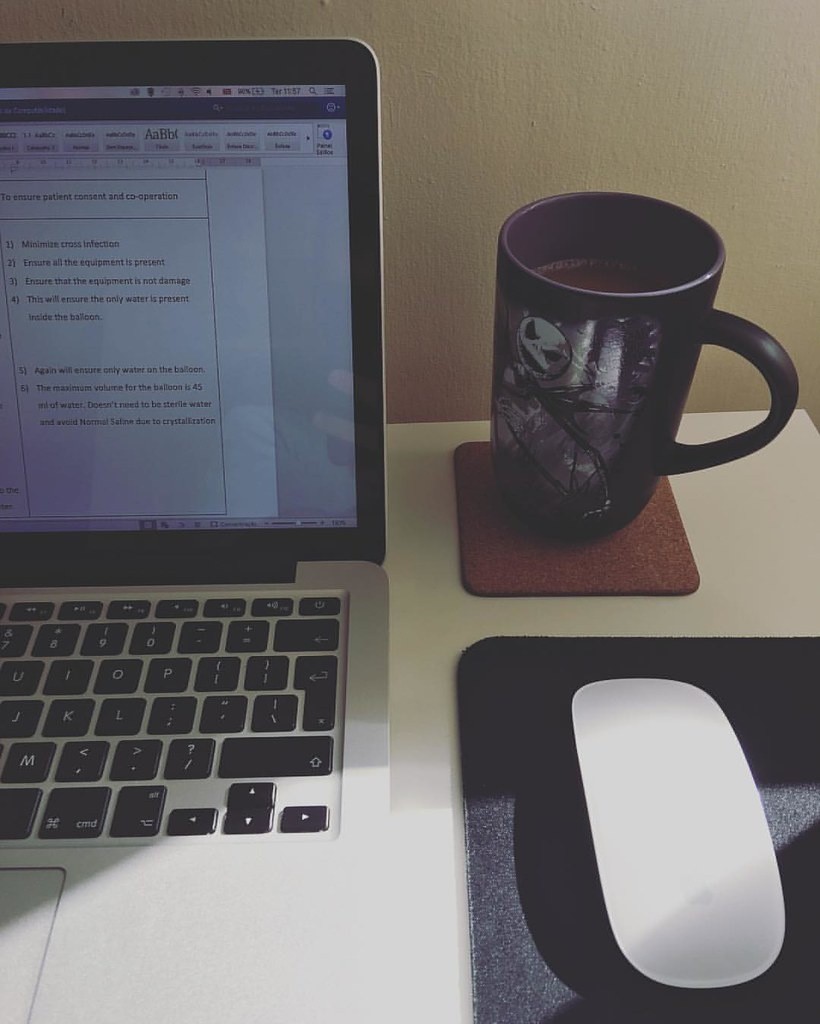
[0,405,820,1022]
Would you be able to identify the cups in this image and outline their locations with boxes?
[489,190,798,537]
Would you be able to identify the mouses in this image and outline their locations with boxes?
[571,677,785,989]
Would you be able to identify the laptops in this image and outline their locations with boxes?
[0,38,391,1024]
